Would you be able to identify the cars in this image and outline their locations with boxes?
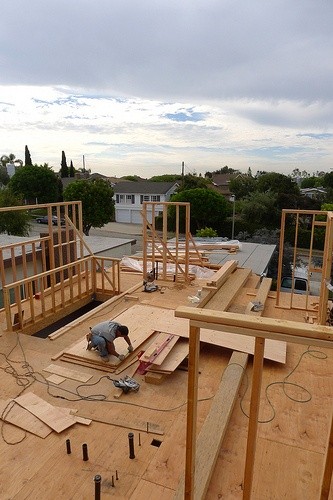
[36,215,57,225]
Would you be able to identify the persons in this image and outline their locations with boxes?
[86,321,134,362]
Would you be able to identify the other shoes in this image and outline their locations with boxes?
[101,355,109,362]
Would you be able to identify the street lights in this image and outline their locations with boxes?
[230,194,236,240]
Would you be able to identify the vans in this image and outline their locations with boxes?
[280,272,312,296]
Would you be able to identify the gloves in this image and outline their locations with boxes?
[127,345,133,353]
[118,354,125,361]
